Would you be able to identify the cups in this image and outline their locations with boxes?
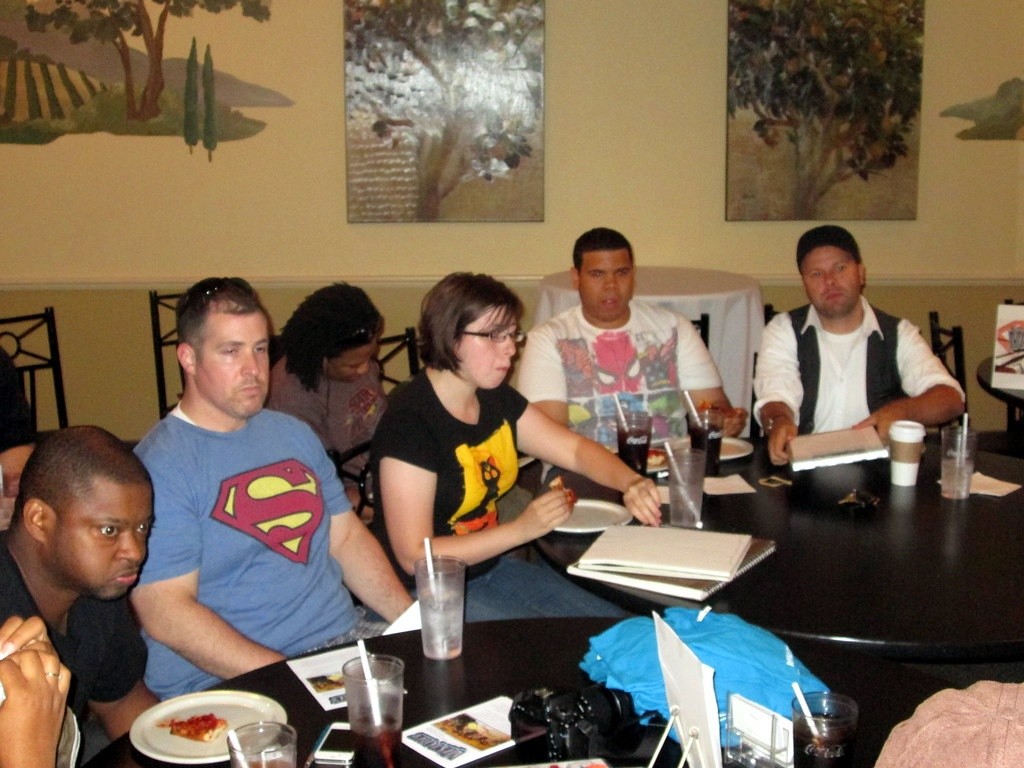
[940,425,977,500]
[342,654,404,768]
[414,555,467,661]
[888,420,927,486]
[617,410,650,474]
[667,448,708,528]
[686,410,725,472]
[226,721,298,767]
[791,691,858,768]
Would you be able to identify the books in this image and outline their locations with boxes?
[787,425,890,472]
[567,522,776,601]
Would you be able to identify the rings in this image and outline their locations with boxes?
[47,672,60,678]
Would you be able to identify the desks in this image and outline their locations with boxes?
[536,439,1024,673]
[534,266,766,437]
[77,616,1024,768]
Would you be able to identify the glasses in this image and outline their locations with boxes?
[176,278,253,320]
[463,329,527,344]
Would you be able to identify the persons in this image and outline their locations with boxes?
[0,343,161,768]
[367,269,666,620]
[268,276,387,522]
[121,277,418,699]
[514,227,749,436]
[753,223,965,467]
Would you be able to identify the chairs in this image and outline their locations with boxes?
[328,441,391,555]
[373,327,419,395]
[927,309,1024,457]
[149,290,184,419]
[0,309,67,449]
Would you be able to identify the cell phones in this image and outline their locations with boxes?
[313,721,354,765]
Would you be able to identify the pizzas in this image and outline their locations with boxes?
[169,713,227,742]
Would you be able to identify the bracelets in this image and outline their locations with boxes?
[765,414,786,431]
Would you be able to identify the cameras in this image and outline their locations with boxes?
[512,668,634,761]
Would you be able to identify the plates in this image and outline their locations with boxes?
[553,498,634,534]
[128,690,288,765]
[669,435,754,461]
[646,447,669,474]
